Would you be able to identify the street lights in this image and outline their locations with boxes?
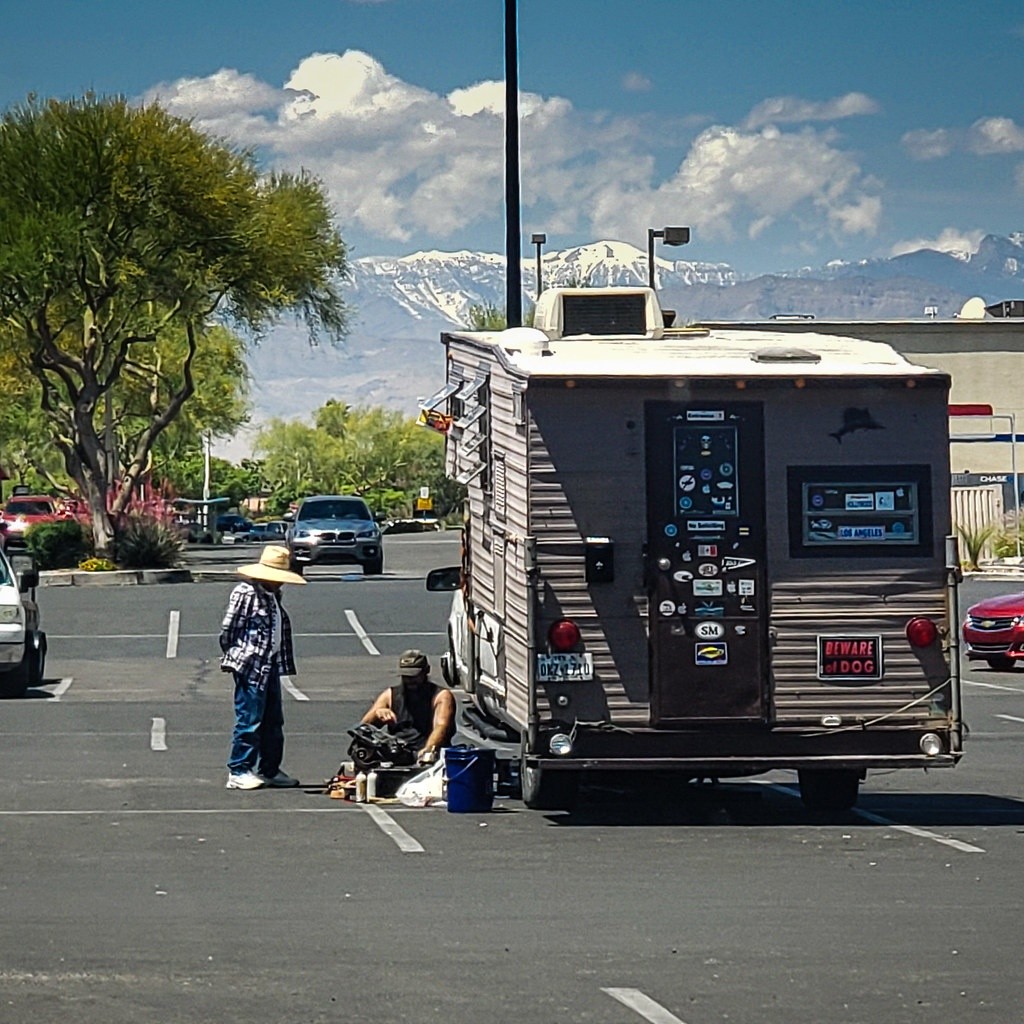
[647,225,691,290]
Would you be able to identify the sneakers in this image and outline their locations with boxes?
[226,770,265,789]
[257,769,300,787]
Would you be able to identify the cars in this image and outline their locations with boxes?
[216,513,291,545]
[962,590,1024,671]
[0,546,47,700]
[0,484,93,556]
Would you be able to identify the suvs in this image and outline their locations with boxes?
[285,494,387,577]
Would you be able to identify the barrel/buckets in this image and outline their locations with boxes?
[442,744,498,813]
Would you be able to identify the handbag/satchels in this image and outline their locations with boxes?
[395,747,451,807]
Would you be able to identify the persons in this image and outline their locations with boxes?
[218,545,309,791]
[358,649,457,759]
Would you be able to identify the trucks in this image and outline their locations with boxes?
[415,282,969,815]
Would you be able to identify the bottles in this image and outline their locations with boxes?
[354,769,367,802]
[366,769,378,802]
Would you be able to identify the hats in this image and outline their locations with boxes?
[238,545,307,585]
[395,650,427,676]
